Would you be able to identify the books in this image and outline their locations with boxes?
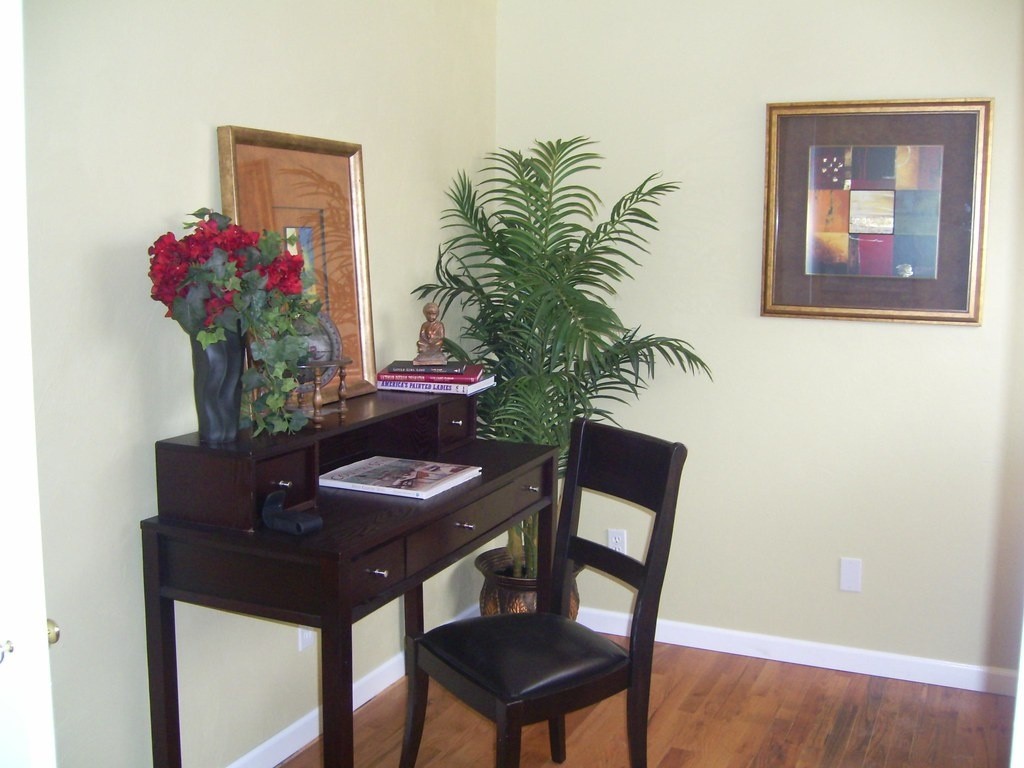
[319,456,482,500]
[388,360,467,374]
[376,365,485,384]
[377,373,498,397]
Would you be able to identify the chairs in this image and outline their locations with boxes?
[398,417,689,768]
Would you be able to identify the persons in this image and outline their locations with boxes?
[416,303,446,355]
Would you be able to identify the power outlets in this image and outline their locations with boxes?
[606,529,628,556]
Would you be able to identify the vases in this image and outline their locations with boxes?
[189,326,244,445]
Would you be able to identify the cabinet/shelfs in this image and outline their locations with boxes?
[137,390,561,768]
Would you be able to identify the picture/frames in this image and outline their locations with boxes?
[216,124,378,422]
[759,97,996,327]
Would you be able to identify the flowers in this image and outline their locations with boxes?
[147,207,324,439]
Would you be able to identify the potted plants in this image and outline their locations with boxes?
[410,134,714,622]
